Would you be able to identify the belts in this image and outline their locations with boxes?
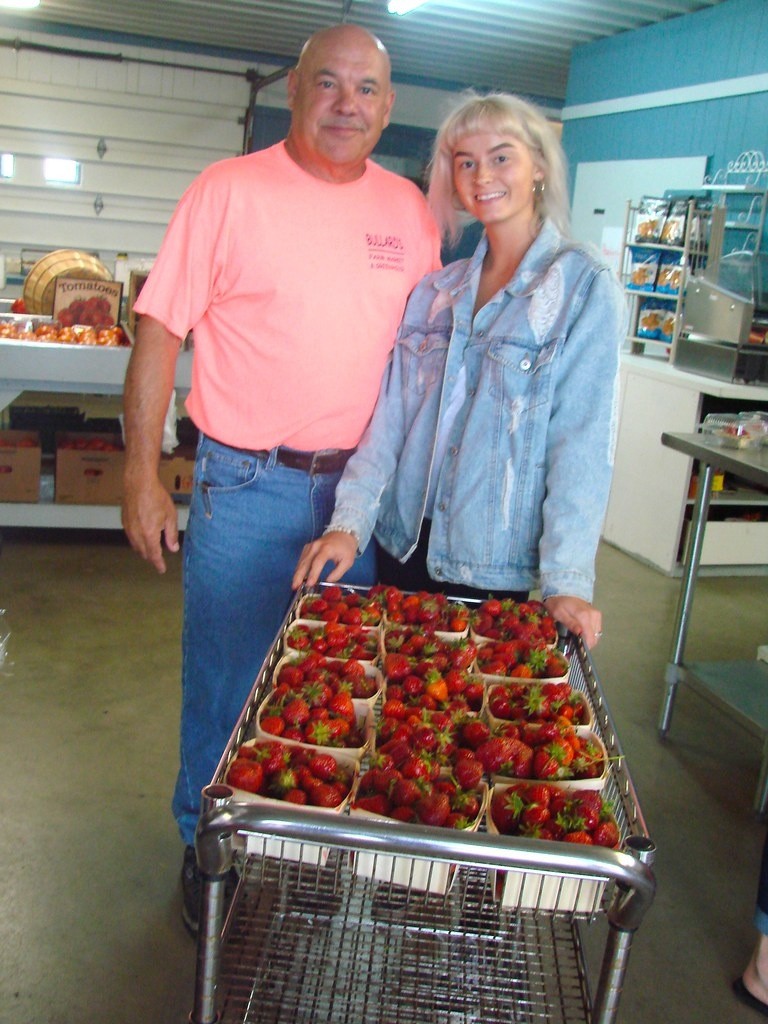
[204,430,357,475]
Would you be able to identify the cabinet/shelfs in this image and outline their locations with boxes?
[611,197,727,370]
[600,346,768,578]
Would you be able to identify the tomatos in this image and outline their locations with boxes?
[0,438,195,477]
[10,297,27,314]
[1,319,130,347]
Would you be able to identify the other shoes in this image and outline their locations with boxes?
[377,877,422,912]
[179,844,241,937]
[461,871,501,948]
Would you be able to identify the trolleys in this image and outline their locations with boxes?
[193,580,656,1023]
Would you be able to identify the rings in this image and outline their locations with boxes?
[595,632,603,637]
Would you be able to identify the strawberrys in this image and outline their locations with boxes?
[226,585,618,844]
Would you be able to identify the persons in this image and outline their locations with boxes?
[292,95,625,652]
[732,818,768,1018]
[122,24,443,931]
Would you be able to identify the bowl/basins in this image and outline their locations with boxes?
[222,593,623,915]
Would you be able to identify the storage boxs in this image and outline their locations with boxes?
[158,450,196,495]
[0,271,196,388]
[220,591,623,918]
[54,430,128,506]
[0,431,42,504]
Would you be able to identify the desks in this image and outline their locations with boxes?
[656,428,768,815]
